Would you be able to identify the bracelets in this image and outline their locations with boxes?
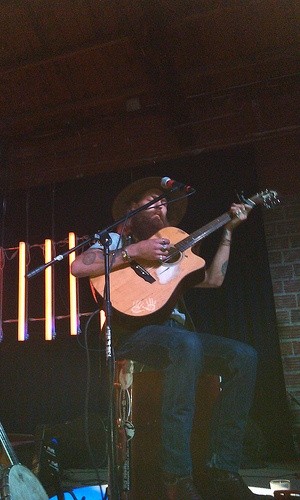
[219,239,232,246]
[121,247,136,263]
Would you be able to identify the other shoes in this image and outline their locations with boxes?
[155,475,204,500]
[205,473,273,500]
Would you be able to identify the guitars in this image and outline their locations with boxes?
[89,189,281,332]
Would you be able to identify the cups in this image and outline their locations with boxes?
[269,479,291,497]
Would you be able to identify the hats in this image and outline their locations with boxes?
[112,177,188,227]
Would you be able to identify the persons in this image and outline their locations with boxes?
[70,176,258,499]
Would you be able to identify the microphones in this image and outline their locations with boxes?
[161,176,194,193]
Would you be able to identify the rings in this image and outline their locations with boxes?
[243,208,245,211]
[238,209,242,214]
[159,254,162,260]
[163,244,166,251]
[162,239,165,245]
[234,210,238,215]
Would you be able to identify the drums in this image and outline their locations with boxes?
[0,465,50,500]
